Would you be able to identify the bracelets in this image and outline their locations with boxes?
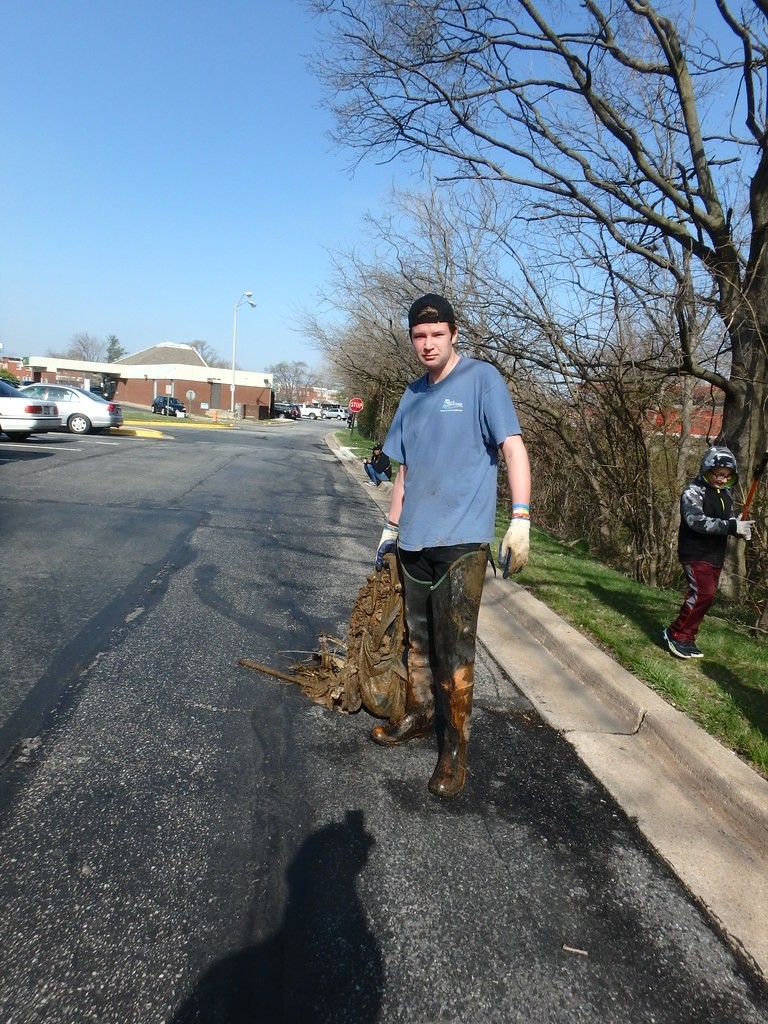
[512,503,529,510]
[510,516,530,521]
[511,512,530,517]
[386,519,399,528]
[512,508,530,515]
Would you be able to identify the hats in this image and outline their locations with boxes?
[372,443,384,450]
[409,293,456,328]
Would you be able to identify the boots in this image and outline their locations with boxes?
[370,658,432,745]
[428,682,488,798]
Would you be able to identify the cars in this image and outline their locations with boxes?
[273,401,349,422]
[152,396,186,416]
[91,386,109,401]
[0,377,61,440]
[18,382,124,434]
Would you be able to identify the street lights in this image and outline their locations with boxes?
[230,291,256,413]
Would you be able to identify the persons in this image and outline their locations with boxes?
[363,443,393,487]
[369,292,533,798]
[661,445,756,659]
[293,406,299,420]
[347,412,353,429]
[285,407,292,418]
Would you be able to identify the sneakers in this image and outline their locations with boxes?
[662,629,692,659]
[686,641,704,657]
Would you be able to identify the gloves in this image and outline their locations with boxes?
[498,504,530,579]
[376,518,398,572]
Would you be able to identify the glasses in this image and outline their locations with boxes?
[709,470,733,481]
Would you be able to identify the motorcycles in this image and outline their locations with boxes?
[347,415,353,427]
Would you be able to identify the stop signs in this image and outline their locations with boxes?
[349,398,363,413]
